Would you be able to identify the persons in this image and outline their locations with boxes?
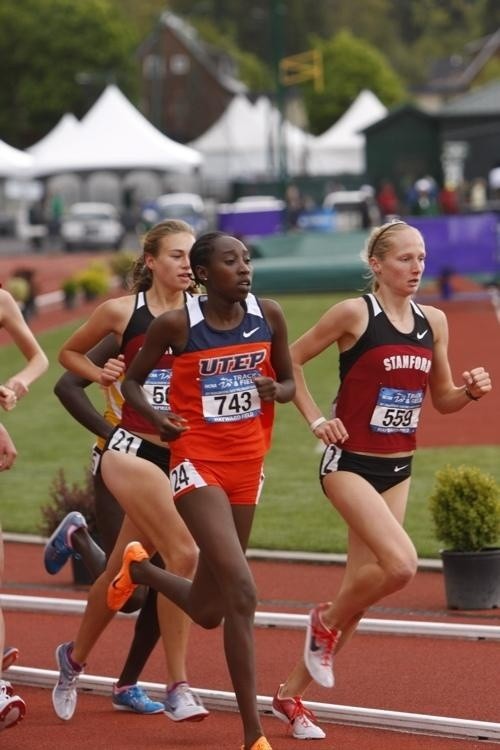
[1,218,211,728]
[49,168,498,222]
[269,216,495,743]
[106,230,292,750]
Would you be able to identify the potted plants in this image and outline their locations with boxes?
[34,457,119,590]
[57,251,140,314]
[425,462,499,613]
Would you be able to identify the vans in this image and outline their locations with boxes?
[323,188,383,230]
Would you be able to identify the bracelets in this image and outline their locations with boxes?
[464,386,482,401]
[309,417,327,432]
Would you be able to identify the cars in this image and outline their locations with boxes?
[143,194,210,241]
[56,202,127,252]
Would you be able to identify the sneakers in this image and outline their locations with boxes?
[52,642,85,720]
[304,602,341,689]
[0,647,26,730]
[112,683,165,714]
[106,542,150,611]
[44,512,88,575]
[272,684,326,739]
[165,682,209,722]
